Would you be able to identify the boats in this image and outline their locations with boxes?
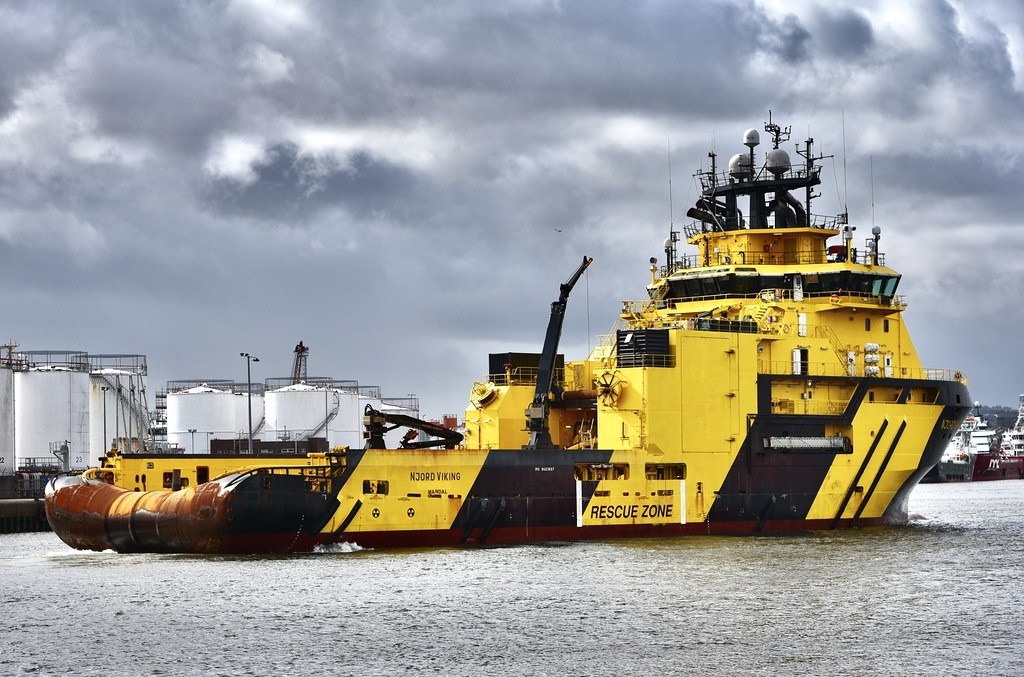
[36,109,982,559]
[918,393,1024,483]
